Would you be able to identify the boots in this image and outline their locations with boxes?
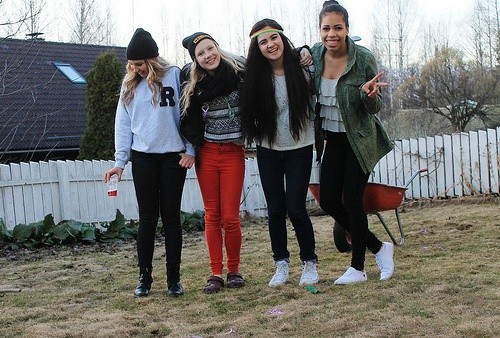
[166,260,184,297]
[134,265,153,298]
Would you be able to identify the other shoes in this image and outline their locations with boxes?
[334,266,367,285]
[298,259,321,288]
[374,242,394,282]
[203,275,224,293]
[268,260,289,289]
[227,272,244,288]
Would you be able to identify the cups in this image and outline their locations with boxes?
[106,175,118,196]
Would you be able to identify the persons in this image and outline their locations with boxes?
[104,27,196,296]
[302,0,395,285]
[180,32,314,293]
[244,19,320,287]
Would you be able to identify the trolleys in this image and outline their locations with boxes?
[308,167,428,254]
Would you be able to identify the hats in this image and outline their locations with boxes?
[183,32,220,63]
[126,28,159,60]
